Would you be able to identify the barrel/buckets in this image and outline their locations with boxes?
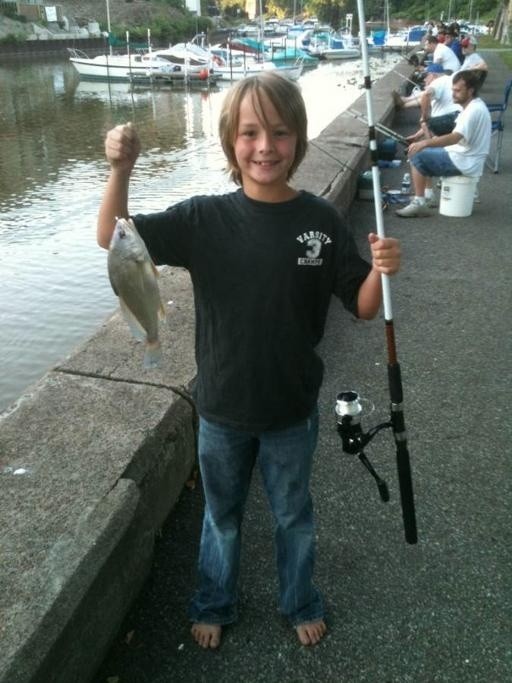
[439,176,480,217]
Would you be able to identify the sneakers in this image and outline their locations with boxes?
[425,195,440,208]
[392,89,405,112]
[395,199,434,219]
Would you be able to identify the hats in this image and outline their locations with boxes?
[460,36,477,47]
[418,63,446,75]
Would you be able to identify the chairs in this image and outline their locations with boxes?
[486,79,512,173]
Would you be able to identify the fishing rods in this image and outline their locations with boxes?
[330,0,426,546]
[305,28,427,213]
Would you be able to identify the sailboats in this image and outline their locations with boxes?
[68,0,173,79]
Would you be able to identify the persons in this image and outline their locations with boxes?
[388,12,489,142]
[93,66,403,655]
[395,69,493,220]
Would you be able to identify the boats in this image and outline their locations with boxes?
[141,24,421,82]
